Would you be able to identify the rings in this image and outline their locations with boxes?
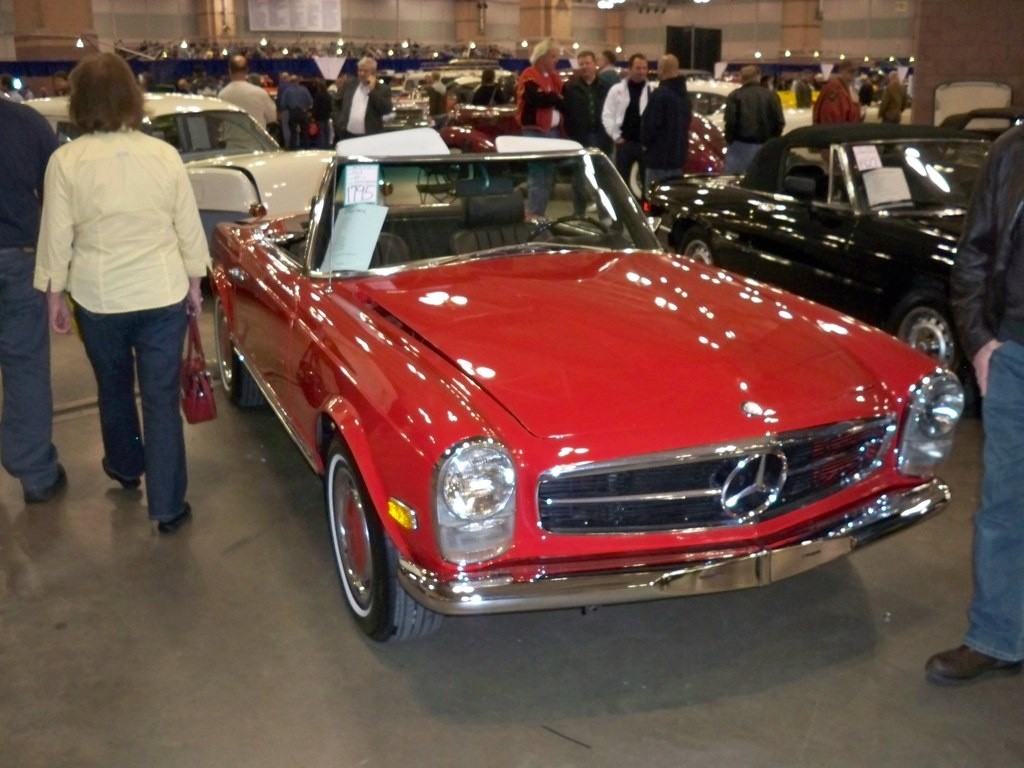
[200,298,203,302]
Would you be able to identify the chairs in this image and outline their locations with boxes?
[373,232,410,268]
[785,165,825,200]
[449,188,554,254]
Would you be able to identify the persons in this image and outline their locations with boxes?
[0,98,68,502]
[923,121,1024,682]
[32,52,212,531]
[723,65,785,176]
[814,60,865,164]
[859,70,907,152]
[729,70,823,109]
[0,54,392,150]
[516,38,692,220]
[472,70,506,105]
[426,71,462,128]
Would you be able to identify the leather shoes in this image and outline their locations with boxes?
[925,644,1024,682]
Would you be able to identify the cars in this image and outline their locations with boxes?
[206,103,965,644]
[640,107,1024,419]
[21,65,912,252]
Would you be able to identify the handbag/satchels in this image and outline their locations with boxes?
[179,307,218,424]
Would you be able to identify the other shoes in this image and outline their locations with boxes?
[22,464,67,504]
[102,457,141,488]
[157,502,192,535]
